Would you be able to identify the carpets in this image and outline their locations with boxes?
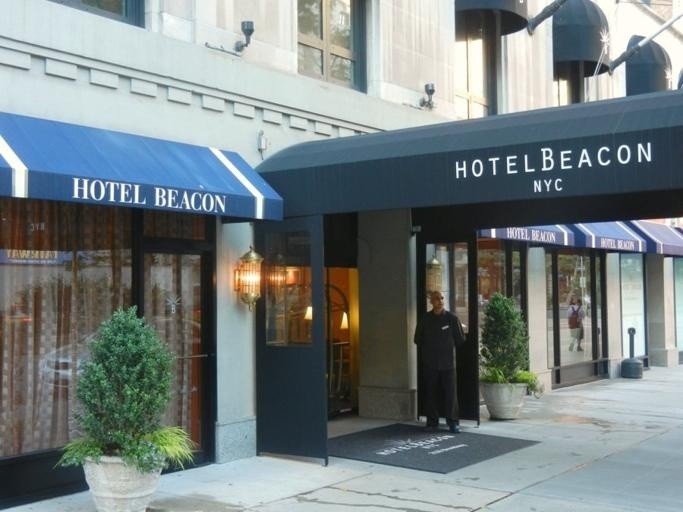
[326,423,541,475]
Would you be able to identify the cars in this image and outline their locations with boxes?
[39,314,201,399]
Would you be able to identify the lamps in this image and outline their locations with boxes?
[419,84,435,108]
[233,22,254,52]
[233,244,263,311]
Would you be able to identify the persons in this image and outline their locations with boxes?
[566,295,585,353]
[412,289,467,437]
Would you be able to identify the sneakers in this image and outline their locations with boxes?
[569,343,583,351]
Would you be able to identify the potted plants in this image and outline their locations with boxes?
[51,303,202,511]
[477,291,545,420]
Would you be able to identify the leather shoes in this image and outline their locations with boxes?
[421,425,436,433]
[449,426,462,432]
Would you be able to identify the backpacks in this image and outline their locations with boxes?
[568,306,580,329]
